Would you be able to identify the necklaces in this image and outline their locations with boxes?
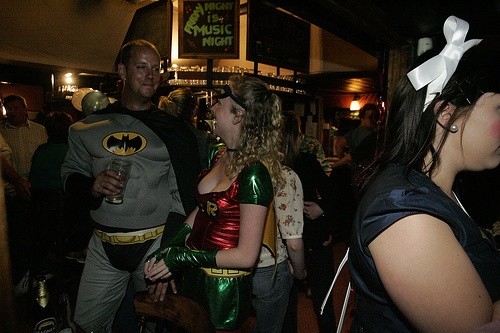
[227,147,242,152]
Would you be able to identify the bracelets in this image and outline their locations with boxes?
[334,162,337,168]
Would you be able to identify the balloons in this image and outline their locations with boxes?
[81,92,110,116]
[71,88,94,111]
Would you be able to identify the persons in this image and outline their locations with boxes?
[35,111,54,126]
[61,39,201,332]
[29,112,87,253]
[250,117,308,332]
[344,39,500,333]
[281,111,338,333]
[332,124,385,227]
[0,95,48,288]
[0,95,32,202]
[142,75,285,333]
[158,87,225,170]
[298,116,332,199]
[344,102,381,150]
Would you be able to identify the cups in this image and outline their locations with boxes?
[105,158,132,205]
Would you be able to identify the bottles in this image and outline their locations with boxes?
[31,276,58,333]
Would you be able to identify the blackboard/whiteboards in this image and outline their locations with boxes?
[177,0,241,60]
[246,0,311,75]
[122,0,173,61]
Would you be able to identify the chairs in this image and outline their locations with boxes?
[130,290,213,333]
